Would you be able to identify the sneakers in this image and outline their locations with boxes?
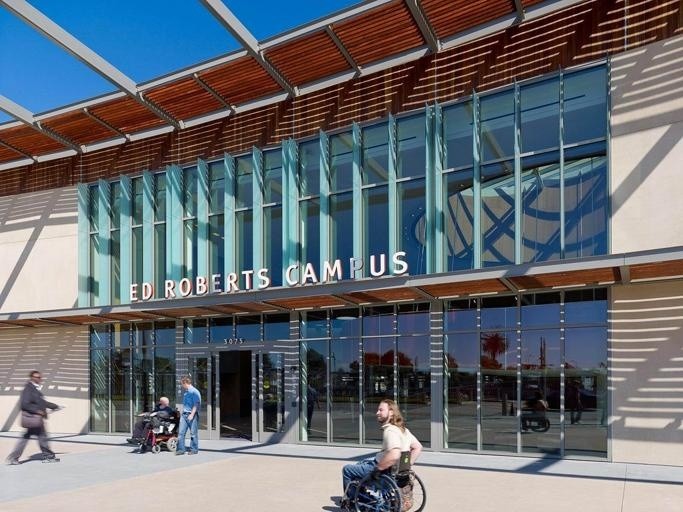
[127,438,137,444]
[42,456,60,463]
[176,451,183,454]
[187,451,194,454]
[136,437,145,444]
[6,454,21,465]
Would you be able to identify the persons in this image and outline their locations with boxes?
[125,396,172,446]
[333,398,423,512]
[3,370,60,465]
[307,382,322,431]
[566,378,586,424]
[173,376,201,455]
[519,389,548,431]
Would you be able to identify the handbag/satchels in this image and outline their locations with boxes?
[21,409,43,430]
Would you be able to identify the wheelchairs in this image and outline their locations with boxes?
[520,409,551,433]
[136,410,181,453]
[341,451,426,512]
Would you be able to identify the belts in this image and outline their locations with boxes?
[183,412,191,414]
